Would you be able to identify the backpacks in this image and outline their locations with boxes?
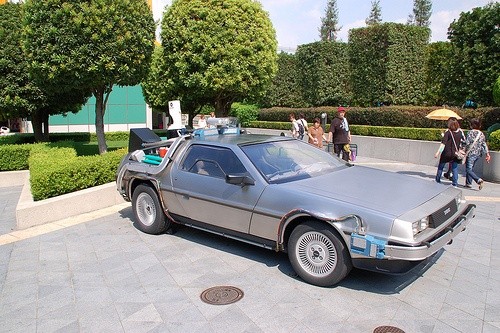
[294,121,304,135]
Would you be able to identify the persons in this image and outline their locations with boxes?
[198,114,206,128]
[327,107,351,161]
[464,118,491,190]
[307,118,327,149]
[434,117,466,188]
[207,112,217,129]
[322,112,327,125]
[288,112,308,140]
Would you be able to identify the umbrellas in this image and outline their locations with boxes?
[424,106,462,131]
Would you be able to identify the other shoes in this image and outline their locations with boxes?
[444,172,453,180]
[479,180,483,190]
[464,185,472,188]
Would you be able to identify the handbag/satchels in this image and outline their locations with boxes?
[454,148,466,164]
[300,119,308,131]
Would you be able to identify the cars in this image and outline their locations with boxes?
[114,126,477,288]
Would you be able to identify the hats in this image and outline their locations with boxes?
[338,106,345,112]
